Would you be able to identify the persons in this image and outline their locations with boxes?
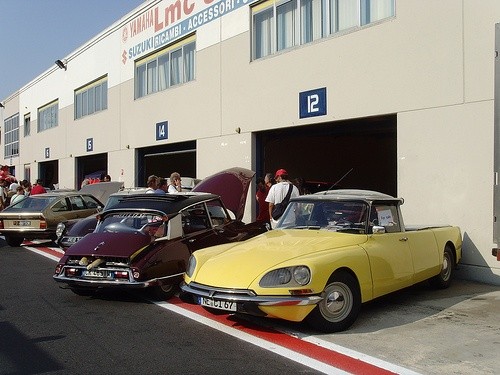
[264,172,276,188]
[255,177,271,220]
[0,179,48,235]
[144,172,183,238]
[269,169,301,230]
[81,174,111,190]
[292,175,314,226]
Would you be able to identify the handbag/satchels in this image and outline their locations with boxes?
[272,183,293,219]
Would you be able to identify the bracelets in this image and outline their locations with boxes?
[270,219,272,221]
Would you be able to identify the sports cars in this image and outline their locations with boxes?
[54,189,152,254]
[0,181,125,246]
[51,167,274,302]
[179,188,464,333]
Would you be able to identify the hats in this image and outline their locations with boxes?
[274,167,289,178]
